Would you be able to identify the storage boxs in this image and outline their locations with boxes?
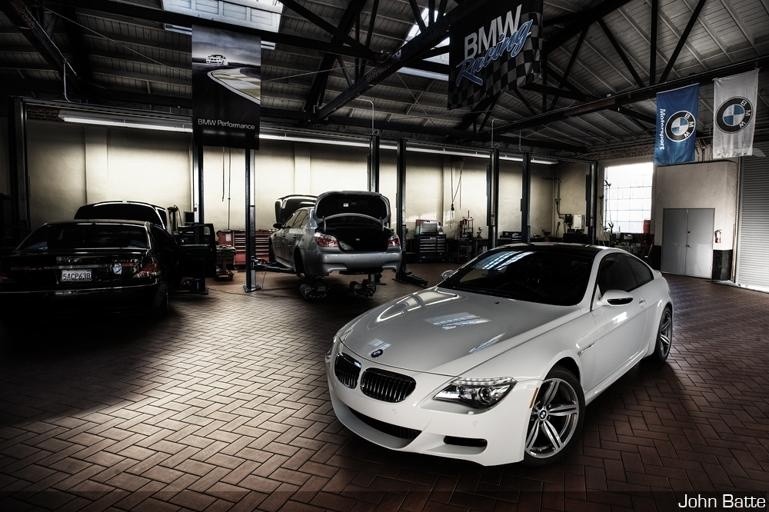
[447,235,487,262]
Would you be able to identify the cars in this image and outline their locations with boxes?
[268,191,402,278]
[1,220,178,321]
[324,242,674,467]
[74,201,217,279]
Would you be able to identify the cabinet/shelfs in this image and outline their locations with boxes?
[498,230,522,243]
[217,228,274,268]
[407,219,447,261]
